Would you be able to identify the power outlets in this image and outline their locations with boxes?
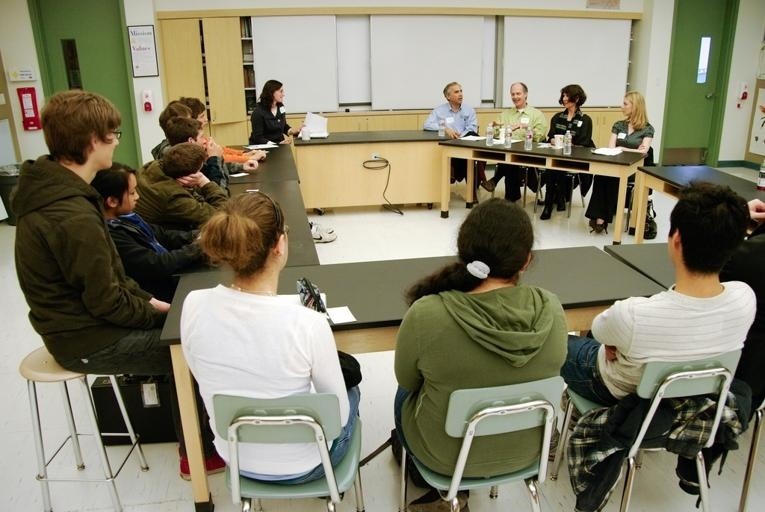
[370,153,381,161]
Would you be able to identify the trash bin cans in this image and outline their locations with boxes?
[0,164,24,226]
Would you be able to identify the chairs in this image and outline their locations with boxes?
[212,390,365,512]
[550,347,742,512]
[534,139,596,217]
[627,147,653,232]
[400,376,565,512]
[737,397,765,512]
[491,168,543,208]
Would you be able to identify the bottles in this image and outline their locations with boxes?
[438,115,445,136]
[504,126,512,149]
[486,125,494,147]
[525,127,533,151]
[757,159,765,190]
[563,130,572,155]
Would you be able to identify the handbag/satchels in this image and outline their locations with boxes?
[644,199,657,239]
[294,278,327,313]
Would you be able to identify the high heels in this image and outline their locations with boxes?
[540,206,553,220]
[589,220,595,232]
[594,221,608,234]
[557,198,565,211]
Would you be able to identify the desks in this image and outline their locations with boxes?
[292,130,452,209]
[182,180,319,273]
[438,135,649,246]
[227,144,301,184]
[604,242,700,290]
[159,246,667,512]
[632,164,765,245]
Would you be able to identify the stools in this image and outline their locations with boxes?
[18,345,150,512]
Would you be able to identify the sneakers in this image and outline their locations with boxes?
[451,177,456,184]
[311,233,337,243]
[481,179,495,191]
[561,389,578,430]
[310,221,334,234]
[179,452,227,481]
[548,432,559,461]
[408,489,469,511]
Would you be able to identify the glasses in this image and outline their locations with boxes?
[109,130,122,138]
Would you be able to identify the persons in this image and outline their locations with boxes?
[134,97,266,225]
[537,85,592,220]
[249,80,305,144]
[584,92,655,235]
[92,162,208,305]
[423,82,487,183]
[11,94,227,479]
[481,82,548,203]
[393,198,569,481]
[561,180,757,407]
[181,193,360,486]
[746,198,765,220]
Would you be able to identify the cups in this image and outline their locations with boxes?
[554,135,563,149]
[302,126,311,141]
